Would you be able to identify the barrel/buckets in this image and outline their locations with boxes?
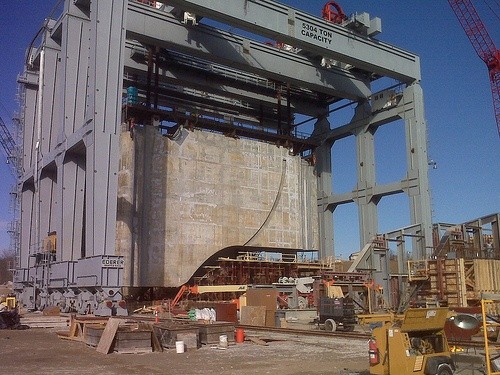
[235,328,244,343]
[175,341,185,354]
[219,335,227,349]
